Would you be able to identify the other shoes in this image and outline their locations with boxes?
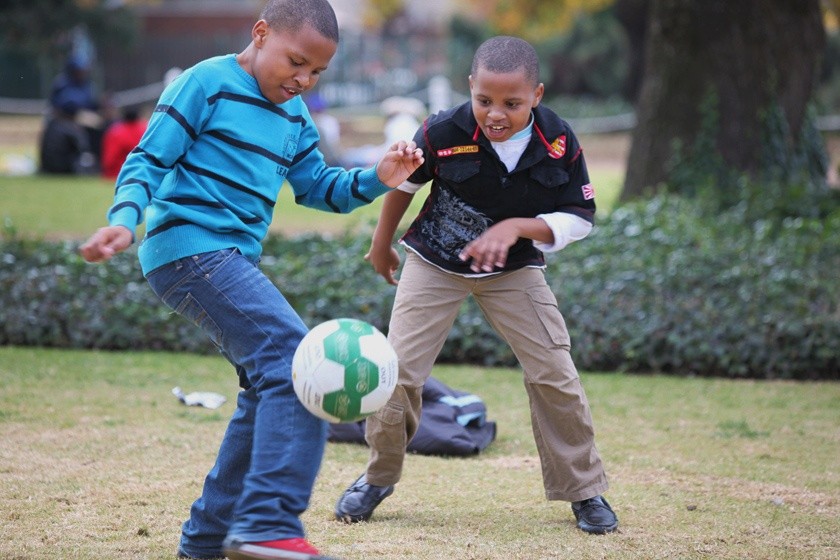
[222,537,320,560]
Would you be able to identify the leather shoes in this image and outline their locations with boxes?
[336,473,394,524]
[571,496,618,534]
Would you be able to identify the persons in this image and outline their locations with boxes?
[334,35,618,534]
[81,0,424,560]
[36,20,153,185]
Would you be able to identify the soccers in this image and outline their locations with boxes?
[291,319,401,423]
